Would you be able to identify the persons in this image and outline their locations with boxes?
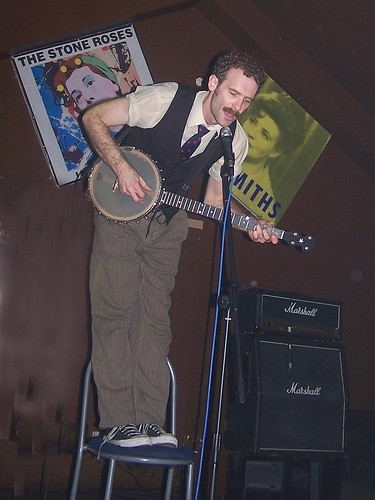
[225,91,306,223]
[82,49,278,447]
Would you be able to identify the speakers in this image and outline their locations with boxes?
[226,333,349,500]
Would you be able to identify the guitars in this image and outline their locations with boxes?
[81,146,316,254]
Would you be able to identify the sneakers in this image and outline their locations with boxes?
[96,423,153,460]
[137,422,178,448]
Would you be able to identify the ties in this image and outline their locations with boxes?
[180,124,211,161]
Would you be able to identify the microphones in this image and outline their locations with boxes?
[220,127,234,176]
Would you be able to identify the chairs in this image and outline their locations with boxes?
[68,358,195,500]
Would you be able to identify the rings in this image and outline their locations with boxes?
[258,235,263,239]
